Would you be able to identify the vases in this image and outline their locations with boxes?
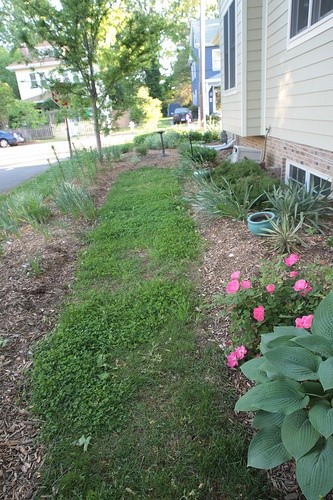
[247,211,276,236]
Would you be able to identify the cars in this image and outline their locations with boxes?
[172,108,193,123]
[0,129,25,148]
